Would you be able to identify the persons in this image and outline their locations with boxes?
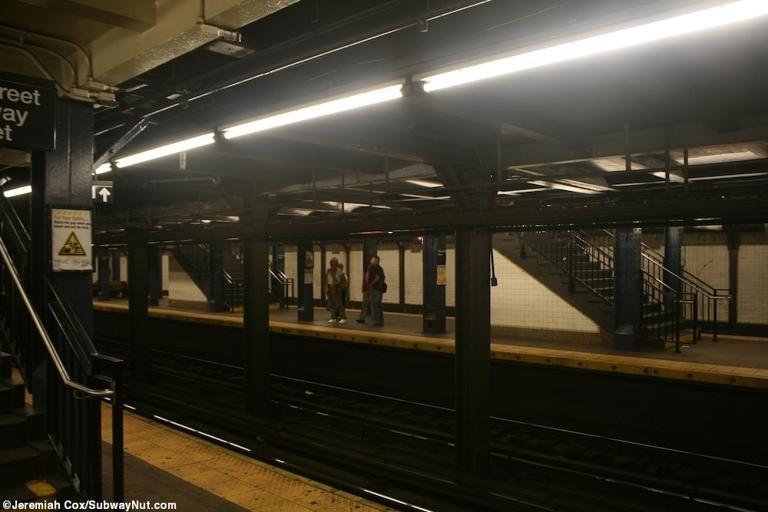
[327,255,386,324]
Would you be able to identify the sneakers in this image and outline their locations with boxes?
[327,317,348,324]
[356,316,384,326]
